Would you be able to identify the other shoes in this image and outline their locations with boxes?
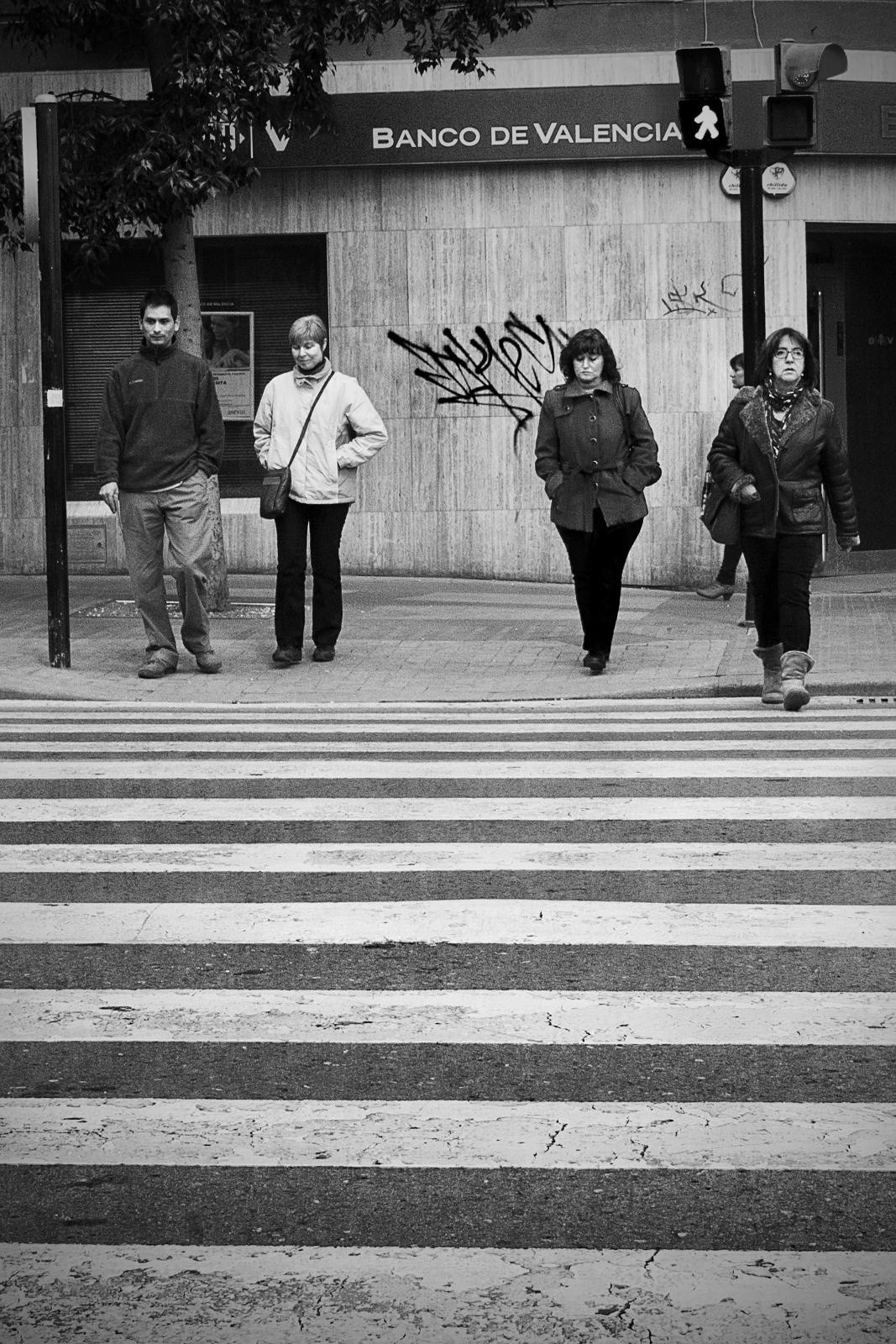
[196,648,222,671]
[583,651,609,675]
[272,644,302,665]
[138,659,176,678]
[312,647,335,661]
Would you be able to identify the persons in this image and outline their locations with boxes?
[696,351,743,600]
[534,328,662,675]
[254,315,389,665]
[94,289,224,680]
[202,314,250,368]
[706,327,861,711]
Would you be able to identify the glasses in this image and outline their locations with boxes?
[775,347,804,360]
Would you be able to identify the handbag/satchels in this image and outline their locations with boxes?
[630,444,662,486]
[260,466,292,519]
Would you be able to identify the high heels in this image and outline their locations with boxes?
[697,580,735,600]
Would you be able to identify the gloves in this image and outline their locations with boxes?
[700,470,742,545]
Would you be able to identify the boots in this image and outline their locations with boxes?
[780,650,815,711]
[753,639,784,702]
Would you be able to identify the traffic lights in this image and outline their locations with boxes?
[675,46,734,149]
[761,95,816,148]
[776,40,848,93]
